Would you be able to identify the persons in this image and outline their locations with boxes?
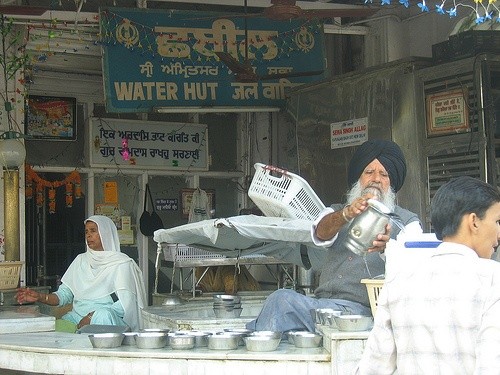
[245,140,423,340]
[358,177,500,375]
[15,215,148,333]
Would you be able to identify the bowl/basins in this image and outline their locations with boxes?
[167,329,283,351]
[88,333,125,348]
[162,297,184,306]
[311,308,372,332]
[136,332,167,349]
[122,332,140,345]
[142,329,170,333]
[288,331,323,348]
[213,294,243,318]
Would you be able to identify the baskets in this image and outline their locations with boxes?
[161,244,227,262]
[0,261,24,289]
[360,279,385,317]
[236,254,267,258]
[248,162,327,221]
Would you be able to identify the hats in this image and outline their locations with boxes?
[347,139,407,193]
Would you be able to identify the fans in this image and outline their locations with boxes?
[212,0,325,82]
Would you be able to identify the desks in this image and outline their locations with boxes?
[176,259,296,301]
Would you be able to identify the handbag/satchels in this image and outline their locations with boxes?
[139,184,163,236]
[187,185,212,224]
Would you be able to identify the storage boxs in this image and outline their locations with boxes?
[247,162,328,221]
[361,278,385,323]
[160,242,227,262]
[0,261,23,290]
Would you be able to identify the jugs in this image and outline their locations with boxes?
[343,198,400,257]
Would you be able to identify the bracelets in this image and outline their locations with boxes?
[44,295,48,304]
[87,315,91,319]
[343,204,353,222]
